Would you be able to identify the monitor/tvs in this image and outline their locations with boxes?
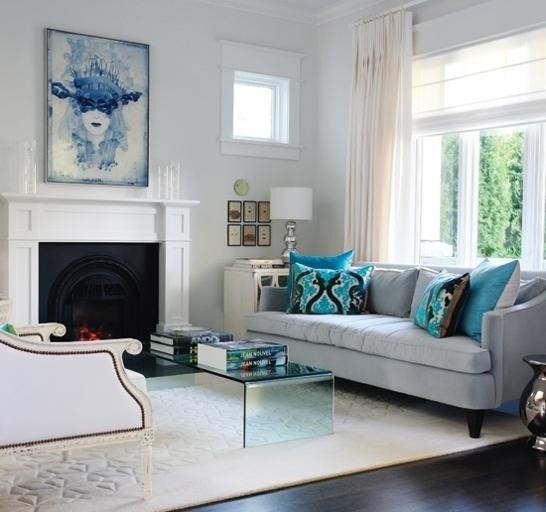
[150,328,288,371]
[233,258,285,268]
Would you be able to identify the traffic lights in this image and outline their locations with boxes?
[460,258,523,343]
[282,248,356,311]
[411,267,473,340]
[287,262,377,316]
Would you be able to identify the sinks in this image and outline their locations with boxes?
[241,260,546,438]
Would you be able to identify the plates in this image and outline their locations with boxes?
[222,266,290,341]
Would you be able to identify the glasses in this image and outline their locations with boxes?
[268,186,314,265]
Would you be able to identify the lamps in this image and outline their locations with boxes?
[0,318,152,512]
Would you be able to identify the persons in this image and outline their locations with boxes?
[50,37,143,171]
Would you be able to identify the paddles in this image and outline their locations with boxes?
[0,367,534,510]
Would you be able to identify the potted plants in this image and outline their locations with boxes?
[258,200,271,223]
[227,224,241,247]
[44,27,152,189]
[258,224,272,247]
[243,223,257,247]
[243,200,257,223]
[227,200,242,223]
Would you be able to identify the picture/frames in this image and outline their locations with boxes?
[520,354,546,453]
